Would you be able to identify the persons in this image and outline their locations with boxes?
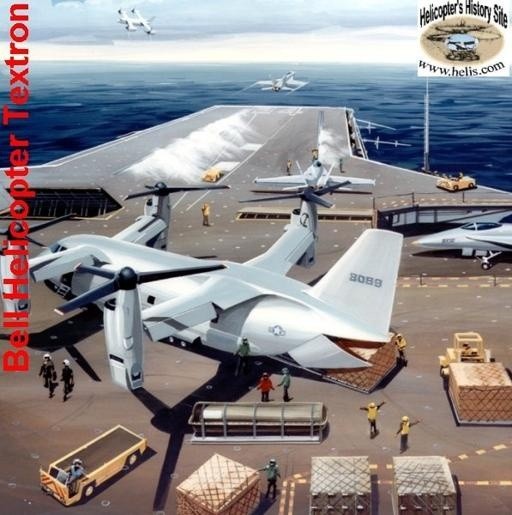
[254,372,275,402]
[395,333,407,367]
[37,354,57,398]
[392,415,425,454]
[358,400,387,441]
[59,358,76,403]
[233,337,251,378]
[275,367,292,402]
[256,459,282,502]
[462,343,473,356]
[201,203,212,227]
[285,159,293,176]
